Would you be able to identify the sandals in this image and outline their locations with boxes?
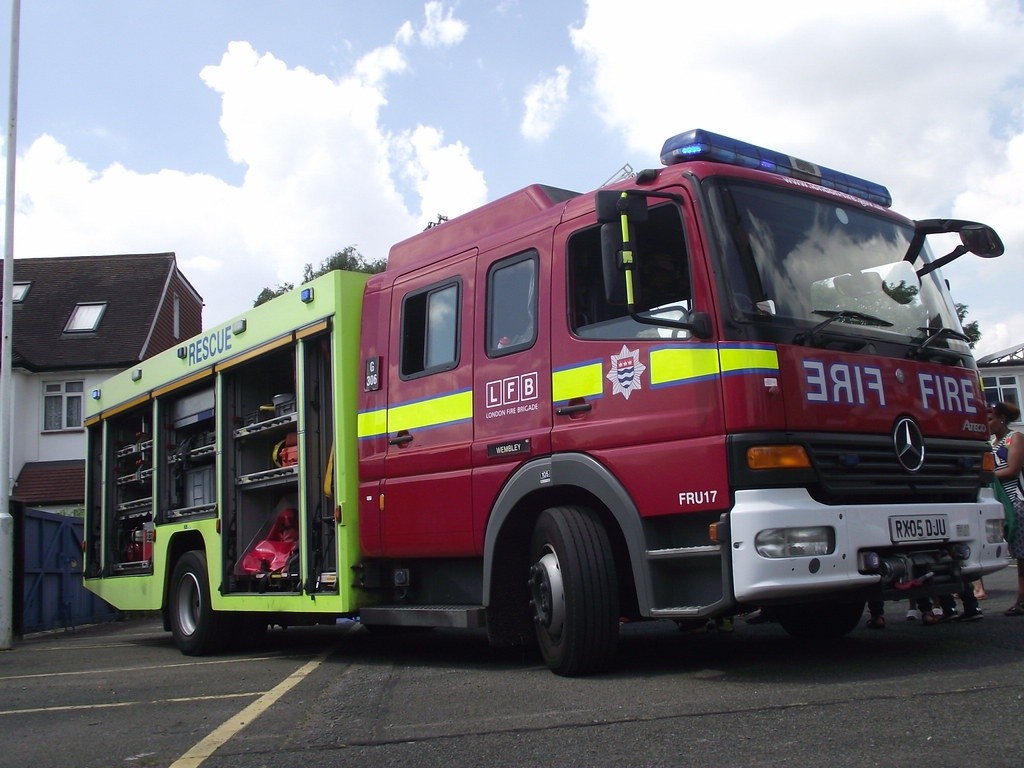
[922,611,935,623]
[866,614,886,629]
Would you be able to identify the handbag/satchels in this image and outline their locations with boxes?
[982,472,1016,543]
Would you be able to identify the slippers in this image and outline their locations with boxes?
[953,592,986,600]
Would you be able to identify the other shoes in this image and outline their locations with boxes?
[1005,600,1024,616]
[906,609,919,620]
[933,607,957,615]
[744,608,775,624]
[690,621,707,633]
[718,618,735,632]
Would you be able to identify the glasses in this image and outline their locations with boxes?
[986,402,998,408]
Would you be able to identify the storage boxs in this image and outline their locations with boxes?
[245,399,298,467]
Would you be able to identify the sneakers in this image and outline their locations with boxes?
[937,607,960,621]
[961,607,984,622]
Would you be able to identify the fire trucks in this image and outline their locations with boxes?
[81,128,1011,679]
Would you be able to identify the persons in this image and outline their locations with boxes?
[865,576,987,628]
[986,402,1024,616]
